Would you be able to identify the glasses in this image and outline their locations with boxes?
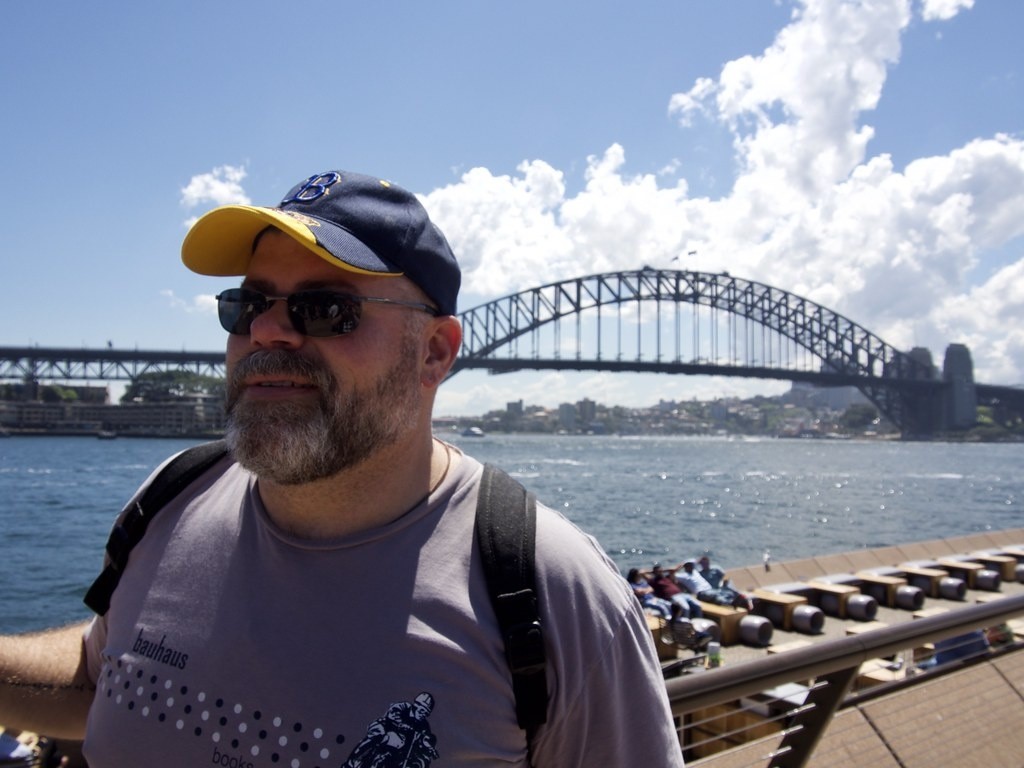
[215,277,437,338]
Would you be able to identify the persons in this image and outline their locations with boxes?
[905,622,1015,681]
[0,167,684,768]
[626,556,751,656]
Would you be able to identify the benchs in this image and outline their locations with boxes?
[644,549,1024,692]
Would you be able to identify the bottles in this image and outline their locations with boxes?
[708,641,720,668]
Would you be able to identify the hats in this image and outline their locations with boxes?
[180,170,461,315]
